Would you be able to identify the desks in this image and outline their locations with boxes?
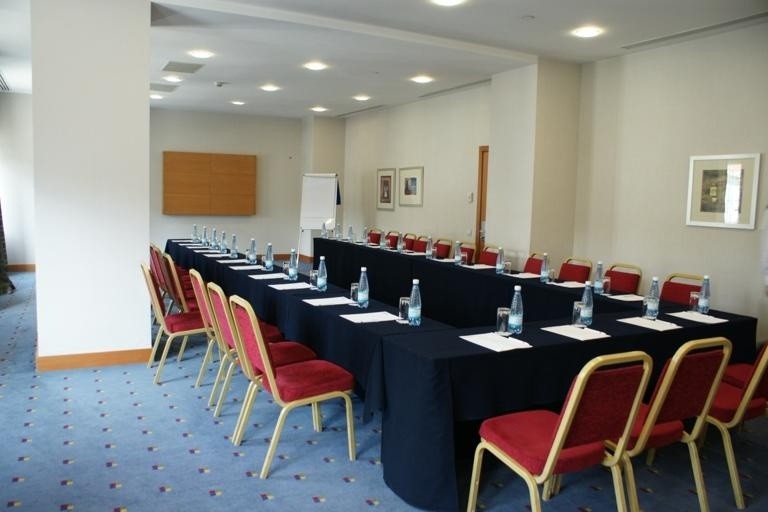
[164,237,457,426]
[377,306,759,511]
[312,235,692,329]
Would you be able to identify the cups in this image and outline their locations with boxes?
[548,268,555,282]
[504,259,511,274]
[689,291,699,311]
[462,252,467,265]
[496,308,509,334]
[385,239,390,249]
[603,275,612,294]
[366,236,371,247]
[402,241,406,253]
[573,300,582,324]
[284,261,290,276]
[325,231,329,239]
[398,297,409,322]
[432,246,437,259]
[642,296,658,320]
[262,255,266,267]
[309,269,318,290]
[246,251,251,263]
[350,283,359,304]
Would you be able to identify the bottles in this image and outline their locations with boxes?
[496,247,504,274]
[698,274,710,314]
[358,266,369,309]
[265,242,273,270]
[426,236,432,259]
[202,224,207,247]
[408,278,422,328]
[288,248,297,281]
[582,280,593,325]
[211,228,217,249]
[221,230,227,254]
[321,222,326,239]
[193,223,198,242]
[647,277,659,317]
[397,232,403,254]
[230,234,238,258]
[455,239,461,264]
[509,284,523,334]
[363,225,367,246]
[380,228,385,249]
[540,252,548,283]
[593,261,604,295]
[249,238,256,263]
[316,255,328,291]
[347,225,353,244]
[336,224,341,242]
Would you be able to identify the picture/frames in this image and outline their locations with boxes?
[685,152,762,230]
[375,167,396,212]
[398,165,424,207]
